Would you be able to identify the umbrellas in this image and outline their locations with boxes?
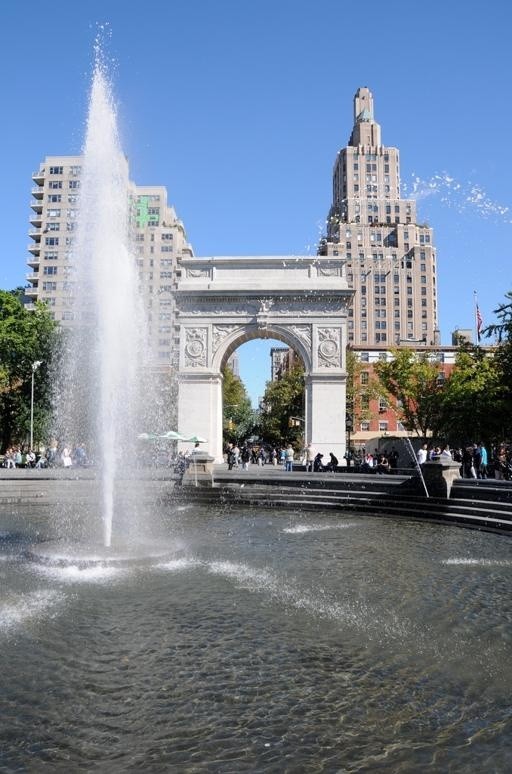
[132,432,207,445]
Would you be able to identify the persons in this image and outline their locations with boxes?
[1,437,200,488]
[416,440,512,482]
[223,438,400,476]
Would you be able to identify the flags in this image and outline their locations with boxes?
[477,305,482,343]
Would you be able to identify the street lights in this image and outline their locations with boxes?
[30,360,43,450]
